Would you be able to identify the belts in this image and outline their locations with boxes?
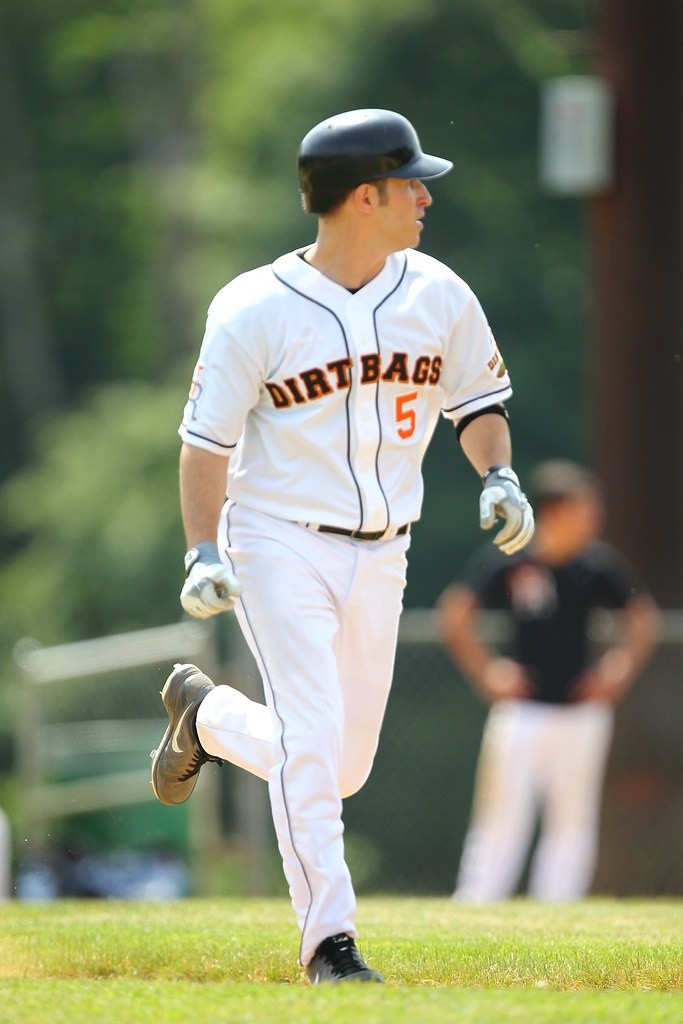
[306,522,408,540]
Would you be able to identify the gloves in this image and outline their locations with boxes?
[479,464,535,556]
[180,540,243,618]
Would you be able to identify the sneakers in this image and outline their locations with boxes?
[152,663,223,805]
[306,933,385,985]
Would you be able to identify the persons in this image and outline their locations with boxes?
[431,459,662,906]
[151,109,535,989]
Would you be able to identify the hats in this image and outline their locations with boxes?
[297,108,455,195]
